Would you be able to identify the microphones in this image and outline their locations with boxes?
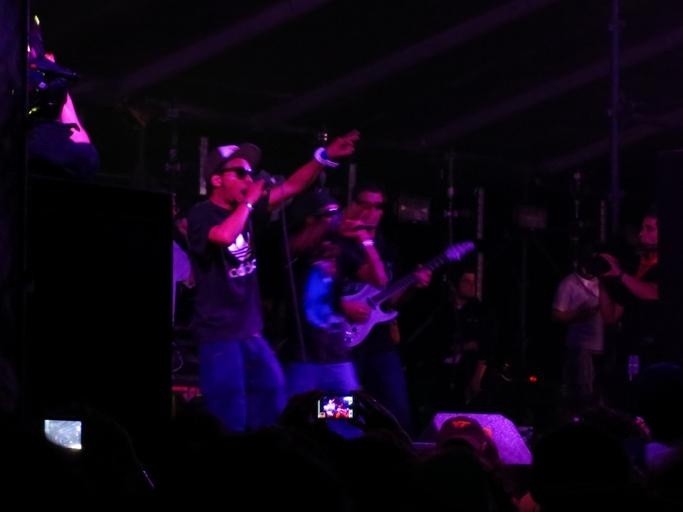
[265,175,284,188]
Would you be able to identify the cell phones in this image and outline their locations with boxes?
[315,396,356,420]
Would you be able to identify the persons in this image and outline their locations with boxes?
[0,130,683,512]
[4,20,100,175]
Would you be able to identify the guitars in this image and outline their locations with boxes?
[321,241,475,350]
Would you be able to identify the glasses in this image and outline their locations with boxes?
[355,199,384,210]
[320,208,340,218]
[223,167,254,180]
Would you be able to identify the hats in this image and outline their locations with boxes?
[203,143,263,182]
[302,190,339,217]
